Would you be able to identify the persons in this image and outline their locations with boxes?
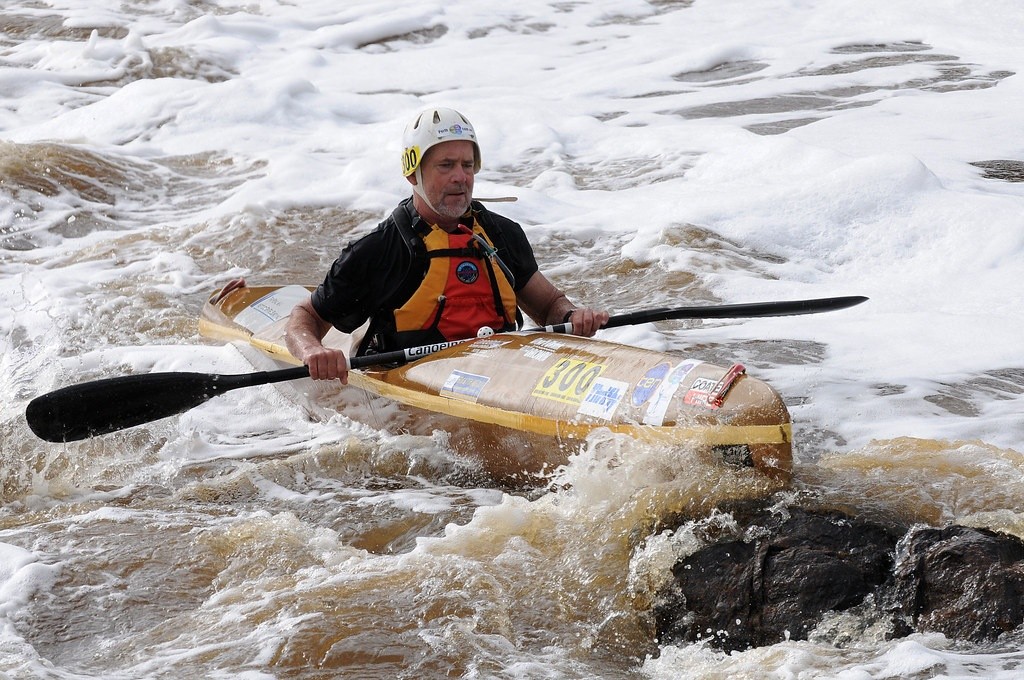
[284,107,608,384]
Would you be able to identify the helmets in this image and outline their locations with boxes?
[401,106,481,177]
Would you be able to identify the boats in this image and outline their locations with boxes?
[197,275,792,495]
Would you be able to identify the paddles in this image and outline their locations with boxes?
[26,294,870,442]
